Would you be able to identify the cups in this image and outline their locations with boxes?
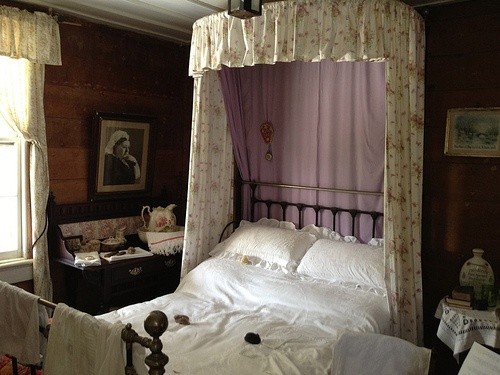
[473,282,497,310]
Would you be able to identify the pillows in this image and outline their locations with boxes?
[209,218,384,295]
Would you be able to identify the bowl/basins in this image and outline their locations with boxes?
[137,226,185,243]
[101,235,127,252]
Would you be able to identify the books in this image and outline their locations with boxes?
[443,286,476,310]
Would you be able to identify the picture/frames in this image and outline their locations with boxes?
[441,107,500,160]
[86,111,155,204]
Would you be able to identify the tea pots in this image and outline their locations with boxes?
[141,203,177,232]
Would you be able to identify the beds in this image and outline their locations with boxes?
[0,169,395,375]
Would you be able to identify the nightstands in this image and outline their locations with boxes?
[434,295,500,364]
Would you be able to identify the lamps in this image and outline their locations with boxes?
[228,0,262,20]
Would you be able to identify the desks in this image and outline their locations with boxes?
[47,194,184,314]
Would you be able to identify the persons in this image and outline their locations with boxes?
[103,131,141,185]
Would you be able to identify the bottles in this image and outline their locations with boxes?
[459,249,495,300]
